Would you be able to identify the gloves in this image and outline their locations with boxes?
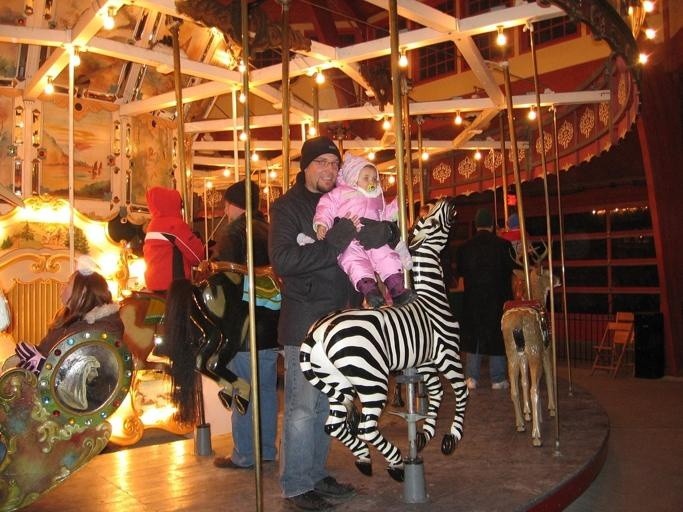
[325,216,358,254]
[356,215,395,250]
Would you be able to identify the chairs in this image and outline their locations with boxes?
[589,311,635,378]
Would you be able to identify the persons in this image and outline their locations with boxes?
[4,267,127,376]
[496,211,532,256]
[56,354,101,410]
[265,135,403,511]
[142,185,209,291]
[310,153,422,309]
[453,207,517,392]
[202,178,281,469]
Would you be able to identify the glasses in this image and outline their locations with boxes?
[311,159,340,171]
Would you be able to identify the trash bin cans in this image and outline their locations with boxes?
[634,312,664,379]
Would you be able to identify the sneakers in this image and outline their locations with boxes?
[466,378,479,390]
[392,289,418,305]
[214,456,253,470]
[491,381,510,390]
[285,491,335,512]
[314,476,357,500]
[366,289,385,308]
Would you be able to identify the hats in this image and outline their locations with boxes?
[300,136,342,173]
[342,157,379,188]
[225,179,259,212]
[474,208,493,226]
